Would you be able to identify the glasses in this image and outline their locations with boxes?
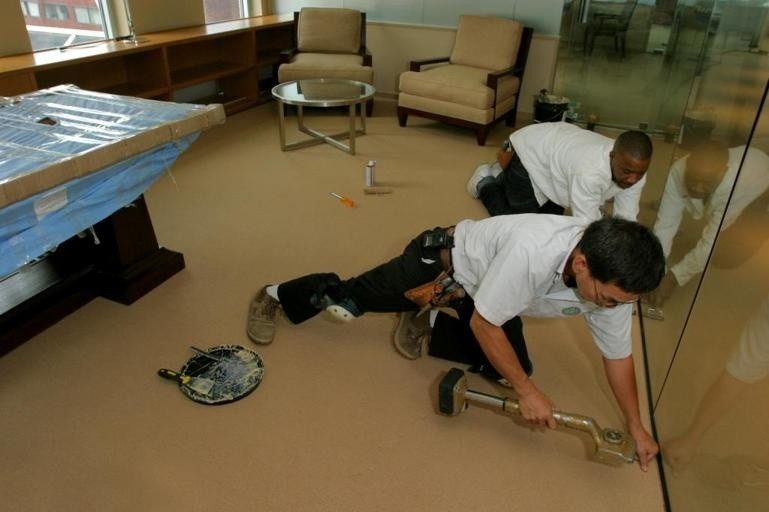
[592,279,642,308]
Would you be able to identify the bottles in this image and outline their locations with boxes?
[586,114,596,132]
[366,159,376,187]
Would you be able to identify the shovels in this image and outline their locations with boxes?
[157,368,215,395]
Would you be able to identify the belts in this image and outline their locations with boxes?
[439,227,456,274]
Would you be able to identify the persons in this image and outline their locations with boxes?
[650,142,769,308]
[465,122,652,221]
[247,211,665,473]
[666,307,765,471]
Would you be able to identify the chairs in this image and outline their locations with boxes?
[279,6,377,118]
[398,14,536,149]
[583,1,638,58]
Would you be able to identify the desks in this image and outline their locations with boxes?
[1,81,227,359]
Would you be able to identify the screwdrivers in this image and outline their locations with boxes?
[330,192,354,208]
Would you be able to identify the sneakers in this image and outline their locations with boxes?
[247,284,282,345]
[467,161,504,199]
[394,308,432,359]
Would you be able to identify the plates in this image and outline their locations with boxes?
[177,343,265,406]
